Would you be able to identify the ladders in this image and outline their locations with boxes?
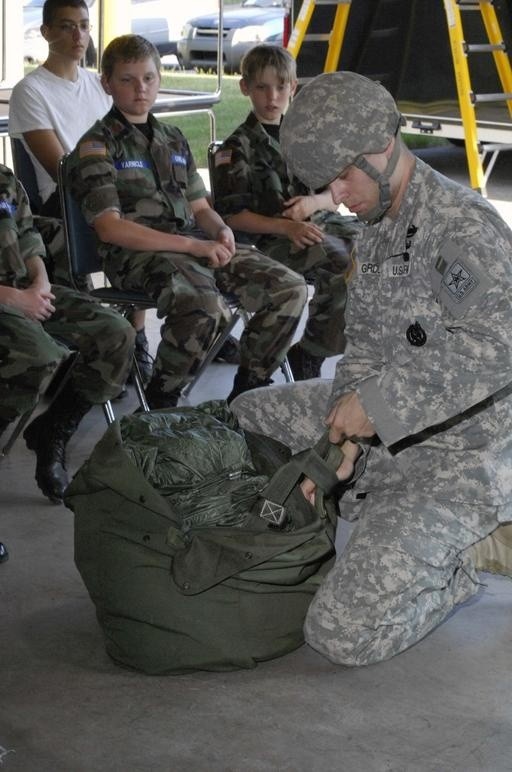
[285,0,512,199]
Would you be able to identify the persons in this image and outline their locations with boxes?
[212,41,374,379]
[224,64,512,665]
[1,162,140,510]
[73,33,309,410]
[8,0,116,212]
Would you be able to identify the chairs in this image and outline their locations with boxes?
[0,124,63,221]
[202,135,315,388]
[52,148,296,439]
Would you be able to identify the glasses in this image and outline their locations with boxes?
[50,22,93,33]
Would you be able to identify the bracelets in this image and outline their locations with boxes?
[215,221,232,240]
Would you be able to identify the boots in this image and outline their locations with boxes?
[22,409,79,505]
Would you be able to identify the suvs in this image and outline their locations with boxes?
[24,4,284,79]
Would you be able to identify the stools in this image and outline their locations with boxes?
[2,239,124,480]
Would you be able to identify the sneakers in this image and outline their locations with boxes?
[130,325,326,381]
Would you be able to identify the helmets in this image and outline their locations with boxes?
[277,68,408,194]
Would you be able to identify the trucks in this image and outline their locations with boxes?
[277,1,511,162]
